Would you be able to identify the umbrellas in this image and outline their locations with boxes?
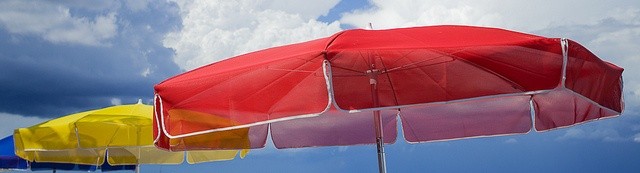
[0,132,138,172]
[13,98,252,172]
[151,23,626,172]
